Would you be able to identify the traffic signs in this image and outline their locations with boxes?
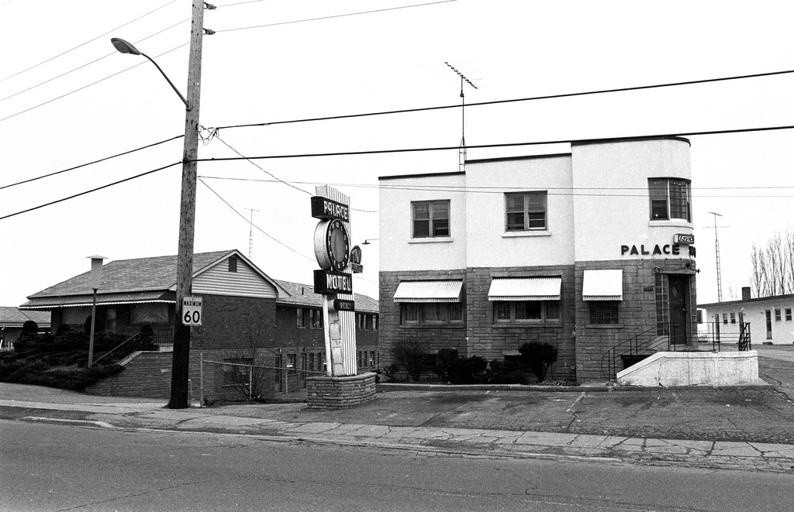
[182,296,202,327]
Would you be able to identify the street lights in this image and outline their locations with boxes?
[110,35,201,411]
